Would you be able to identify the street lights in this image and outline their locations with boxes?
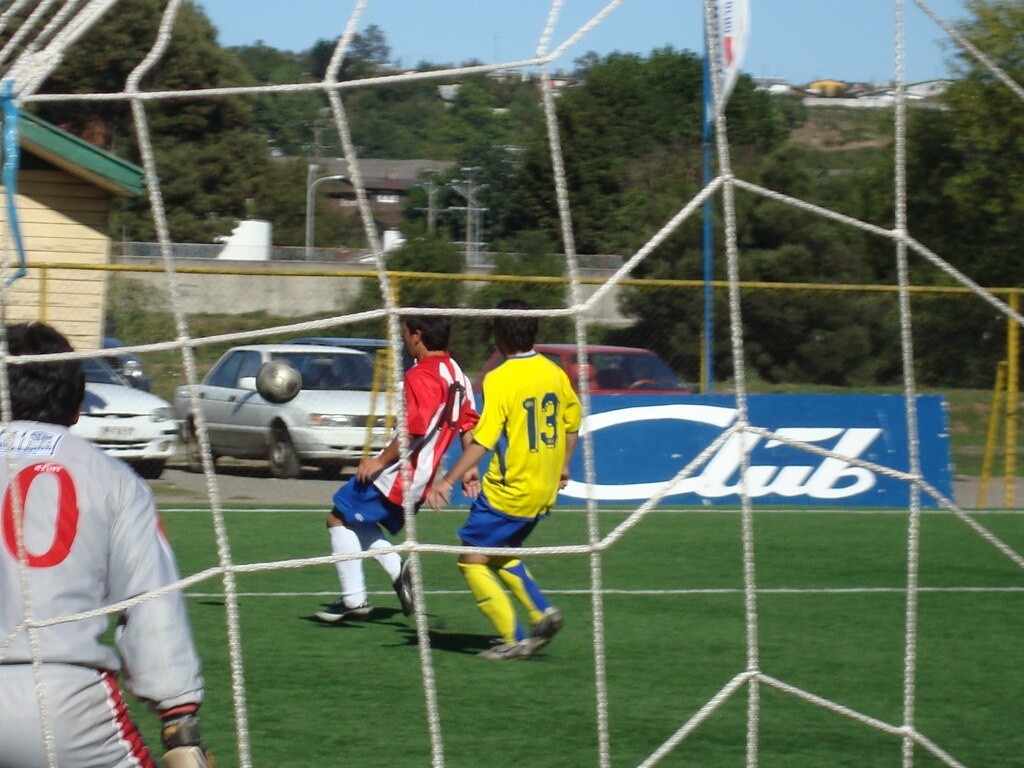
[303,162,347,261]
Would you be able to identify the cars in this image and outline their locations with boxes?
[172,342,414,478]
[66,350,178,480]
[103,337,151,394]
[477,343,699,396]
[281,335,418,393]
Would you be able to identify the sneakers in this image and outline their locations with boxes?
[314,597,371,622]
[475,640,530,659]
[392,558,413,616]
[528,607,563,653]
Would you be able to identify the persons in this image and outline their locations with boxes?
[425,299,581,661]
[1,322,211,768]
[315,299,481,622]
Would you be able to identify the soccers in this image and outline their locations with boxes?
[256,359,303,403]
